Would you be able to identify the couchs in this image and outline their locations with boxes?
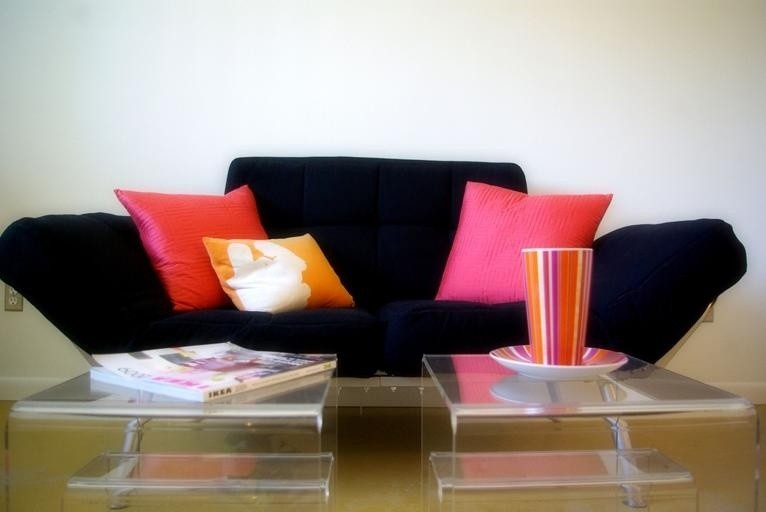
[0,157,746,511]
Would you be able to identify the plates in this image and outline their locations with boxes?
[488,344,629,382]
[491,374,629,408]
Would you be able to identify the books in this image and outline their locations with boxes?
[90,340,337,403]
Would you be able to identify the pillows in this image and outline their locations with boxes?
[113,184,268,312]
[201,233,356,313]
[434,181,613,305]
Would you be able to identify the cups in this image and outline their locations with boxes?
[521,247,594,365]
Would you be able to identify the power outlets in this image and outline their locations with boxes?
[4,282,22,312]
